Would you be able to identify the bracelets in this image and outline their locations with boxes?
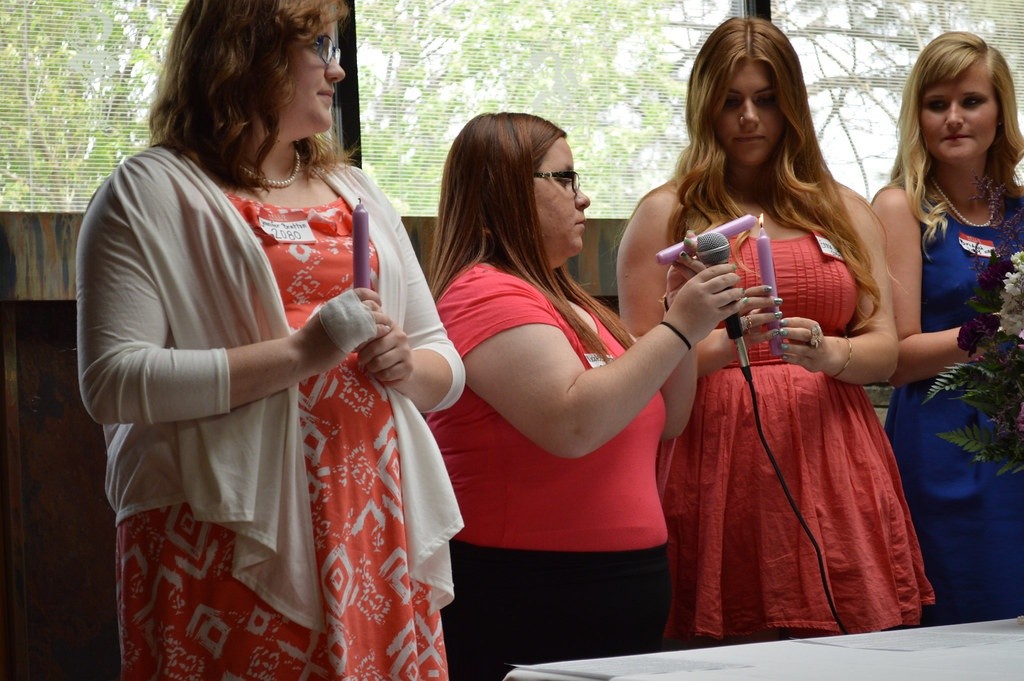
[833,336,853,379]
[658,292,669,314]
[660,322,692,351]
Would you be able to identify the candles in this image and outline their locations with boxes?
[755,213,785,356]
[655,213,762,266]
[352,197,370,289]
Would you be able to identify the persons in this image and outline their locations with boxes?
[872,31,1024,627]
[76,0,466,681]
[421,113,748,681]
[616,16,935,652]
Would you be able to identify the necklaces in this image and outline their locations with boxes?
[930,173,994,227]
[240,148,301,188]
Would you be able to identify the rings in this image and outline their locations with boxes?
[742,315,752,331]
[807,325,822,349]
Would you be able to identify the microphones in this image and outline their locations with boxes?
[697,232,753,381]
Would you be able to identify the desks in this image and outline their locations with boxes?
[503,617,1024,681]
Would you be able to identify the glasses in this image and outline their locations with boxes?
[312,34,341,67]
[533,171,581,193]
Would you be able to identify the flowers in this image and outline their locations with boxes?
[920,168,1024,476]
